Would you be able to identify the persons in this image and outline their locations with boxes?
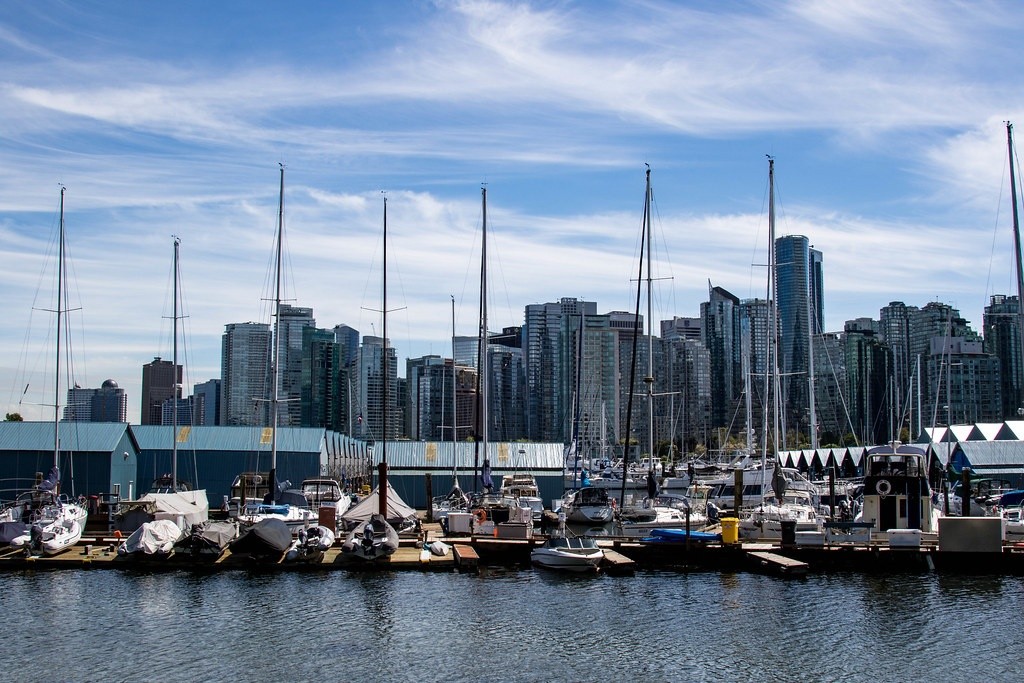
[514,494,520,506]
[612,497,616,510]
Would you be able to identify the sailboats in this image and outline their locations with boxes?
[119,235,211,532]
[337,187,421,531]
[1,181,92,548]
[430,118,1024,525]
[218,161,319,534]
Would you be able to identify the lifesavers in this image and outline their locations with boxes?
[875,479,890,496]
[474,509,486,522]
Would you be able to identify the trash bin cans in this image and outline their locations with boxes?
[720,517,739,543]
[779,519,797,544]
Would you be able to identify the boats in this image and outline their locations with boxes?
[338,514,400,562]
[286,522,334,562]
[431,540,449,556]
[9,515,83,559]
[230,470,292,503]
[227,516,293,560]
[526,525,604,575]
[302,476,352,521]
[171,517,240,563]
[117,515,183,564]
